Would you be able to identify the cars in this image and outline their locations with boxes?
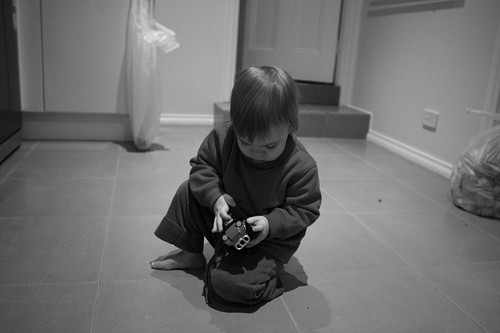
[223,221,251,251]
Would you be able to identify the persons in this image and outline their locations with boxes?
[149,64,323,306]
